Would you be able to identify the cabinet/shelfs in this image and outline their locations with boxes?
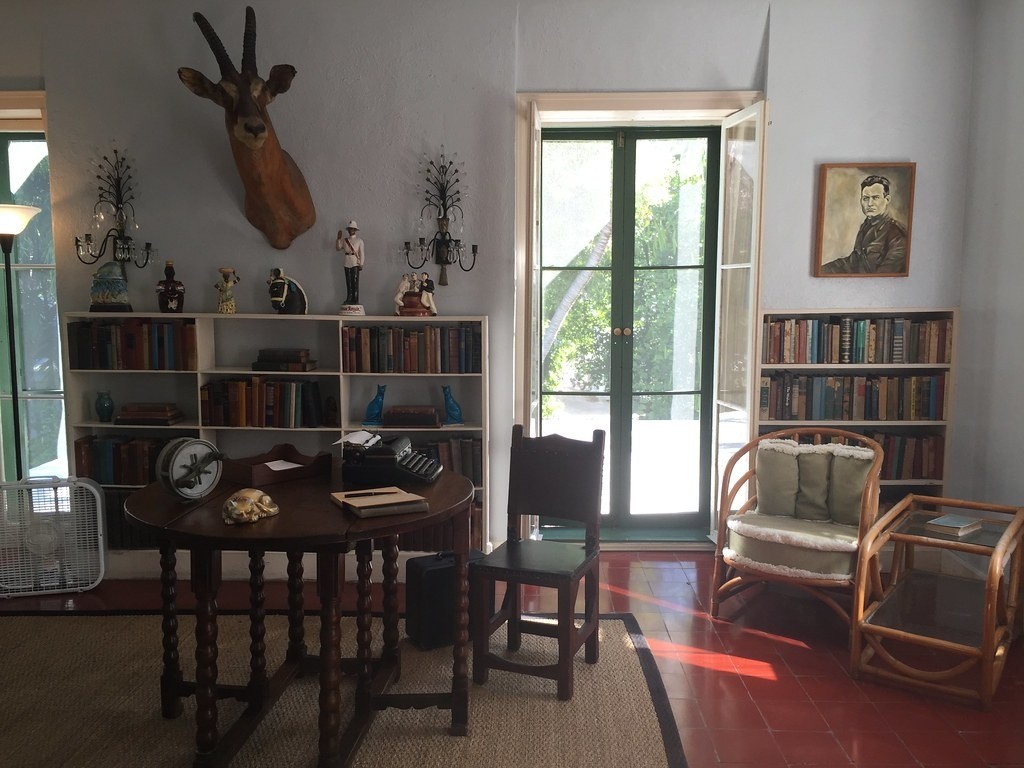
[58,311,489,556]
[752,305,959,541]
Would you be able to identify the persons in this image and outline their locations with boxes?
[214,268,239,313]
[335,220,366,305]
[156,261,185,313]
[393,271,438,316]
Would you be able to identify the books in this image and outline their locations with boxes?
[759,430,944,480]
[382,405,442,428]
[200,375,321,429]
[114,402,185,426]
[252,348,318,372]
[762,315,953,364]
[343,326,482,373]
[878,492,936,541]
[374,497,482,550]
[74,435,168,550]
[760,369,950,421]
[412,437,482,488]
[330,485,429,518]
[924,512,984,537]
[67,319,196,371]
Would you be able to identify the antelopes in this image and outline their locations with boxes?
[175,6,317,251]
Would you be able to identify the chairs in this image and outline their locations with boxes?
[709,427,886,621]
[469,424,606,700]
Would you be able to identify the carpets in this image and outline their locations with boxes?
[0,607,690,768]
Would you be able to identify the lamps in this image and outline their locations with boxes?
[75,136,160,283]
[405,143,480,286]
[1,201,44,555]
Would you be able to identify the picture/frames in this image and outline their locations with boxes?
[815,160,917,278]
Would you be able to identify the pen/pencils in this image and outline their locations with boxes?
[345,492,398,498]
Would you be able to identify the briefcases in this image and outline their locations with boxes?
[405,550,495,652]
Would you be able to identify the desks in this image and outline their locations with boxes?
[849,492,1024,710]
[123,450,475,767]
